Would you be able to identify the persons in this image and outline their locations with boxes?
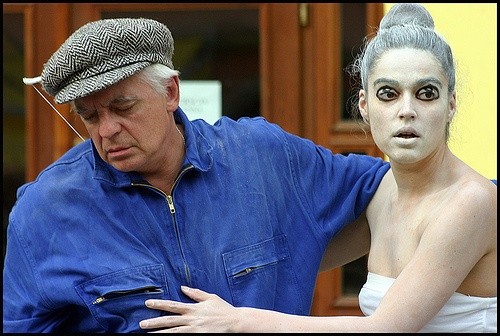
[3,18,496,332]
[139,4,497,333]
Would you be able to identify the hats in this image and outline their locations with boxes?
[42,18,174,104]
